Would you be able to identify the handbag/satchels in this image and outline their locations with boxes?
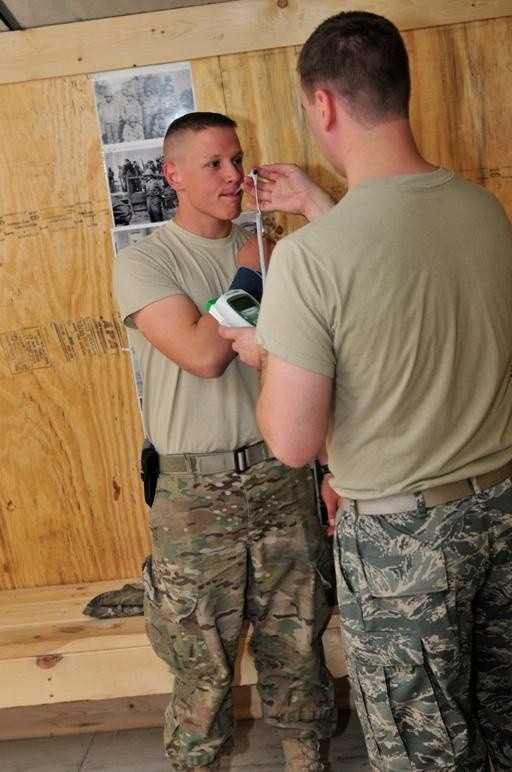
[140,441,158,507]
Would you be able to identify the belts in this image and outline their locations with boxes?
[337,463,510,516]
[159,442,279,474]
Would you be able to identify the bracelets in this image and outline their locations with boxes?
[320,464,332,473]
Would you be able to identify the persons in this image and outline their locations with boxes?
[217,12,511,772]
[112,112,338,771]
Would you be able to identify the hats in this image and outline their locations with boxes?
[83,587,144,618]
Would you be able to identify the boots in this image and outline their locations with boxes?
[283,736,327,771]
